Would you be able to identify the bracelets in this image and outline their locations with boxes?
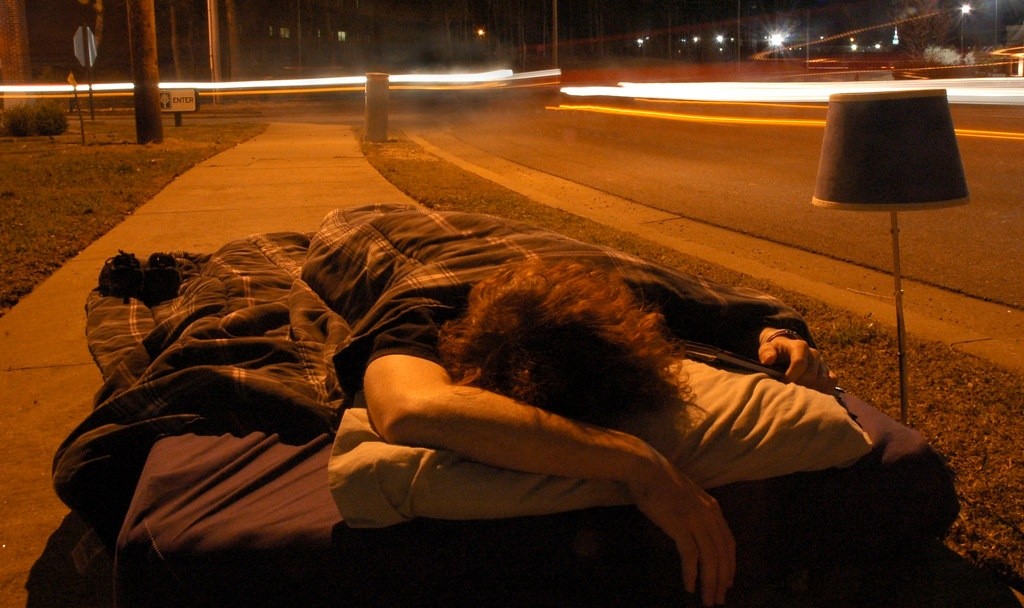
[761,329,804,342]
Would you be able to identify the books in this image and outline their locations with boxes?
[671,338,785,377]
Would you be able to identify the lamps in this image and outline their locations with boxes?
[810,86,970,428]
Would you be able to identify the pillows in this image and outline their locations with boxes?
[326,358,874,531]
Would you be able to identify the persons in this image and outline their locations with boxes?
[364,261,839,608]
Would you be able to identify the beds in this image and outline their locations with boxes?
[50,200,962,607]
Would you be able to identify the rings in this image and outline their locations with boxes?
[820,374,828,383]
